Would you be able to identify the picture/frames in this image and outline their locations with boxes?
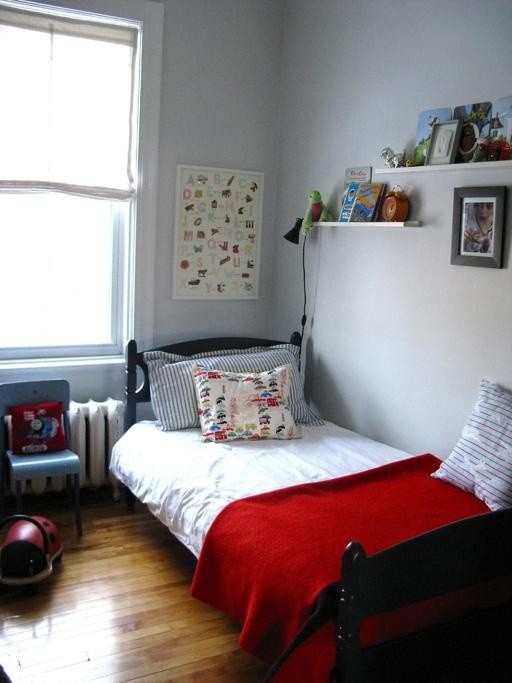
[174,163,265,301]
[426,122,460,165]
[450,186,506,270]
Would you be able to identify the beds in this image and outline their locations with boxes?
[114,331,511,681]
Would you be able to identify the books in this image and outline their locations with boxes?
[340,166,387,222]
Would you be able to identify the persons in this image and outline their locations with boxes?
[463,202,493,253]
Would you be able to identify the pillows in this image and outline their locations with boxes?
[8,399,66,455]
[141,342,329,430]
[427,378,512,511]
[196,366,304,446]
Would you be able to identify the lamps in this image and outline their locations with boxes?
[283,215,310,352]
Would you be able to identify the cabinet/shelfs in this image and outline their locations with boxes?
[306,157,512,230]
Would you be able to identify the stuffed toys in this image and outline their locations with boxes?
[302,189,333,235]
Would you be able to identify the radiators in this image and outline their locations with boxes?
[3,397,127,497]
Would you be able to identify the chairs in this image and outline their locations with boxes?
[0,379,83,539]
